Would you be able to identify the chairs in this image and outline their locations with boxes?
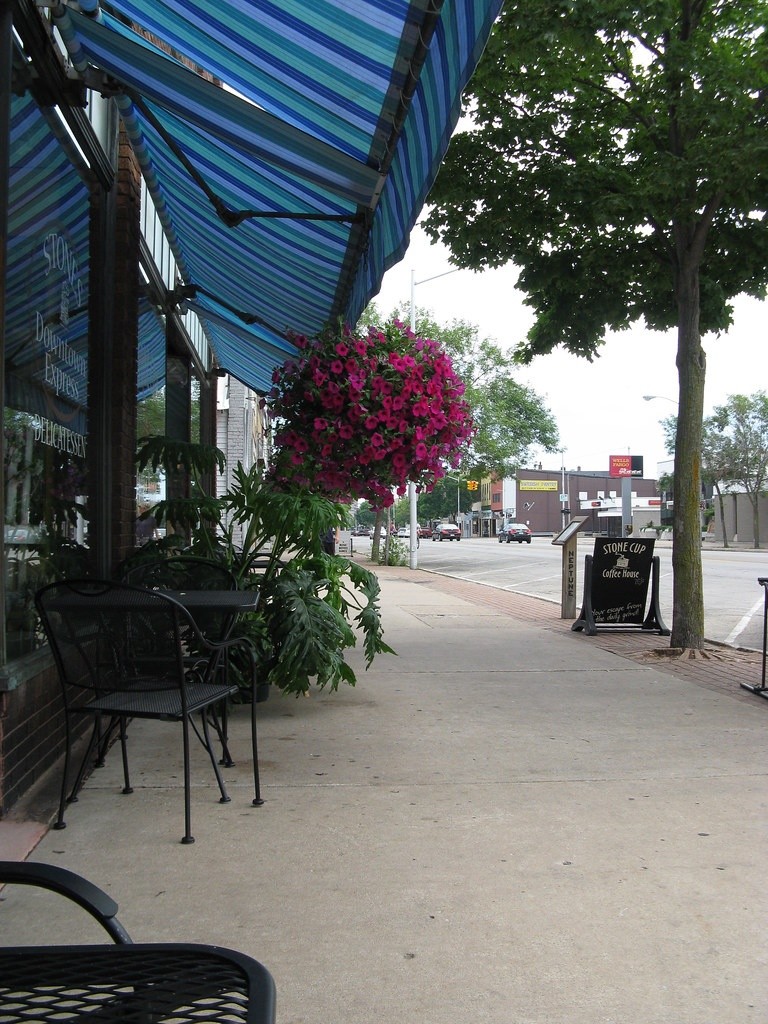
[0,861,152,1024]
[113,558,239,764]
[33,577,263,845]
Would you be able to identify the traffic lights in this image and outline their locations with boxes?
[476,481,478,490]
[467,481,472,490]
[471,481,476,490]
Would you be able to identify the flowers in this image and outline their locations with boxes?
[259,314,477,512]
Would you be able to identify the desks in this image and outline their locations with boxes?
[0,944,276,1024]
[45,589,261,804]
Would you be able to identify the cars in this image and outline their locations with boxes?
[404,528,410,538]
[432,523,461,542]
[417,527,432,538]
[352,529,370,536]
[498,523,531,544]
[397,528,407,538]
[368,527,386,540]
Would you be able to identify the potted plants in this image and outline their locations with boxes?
[127,434,397,705]
